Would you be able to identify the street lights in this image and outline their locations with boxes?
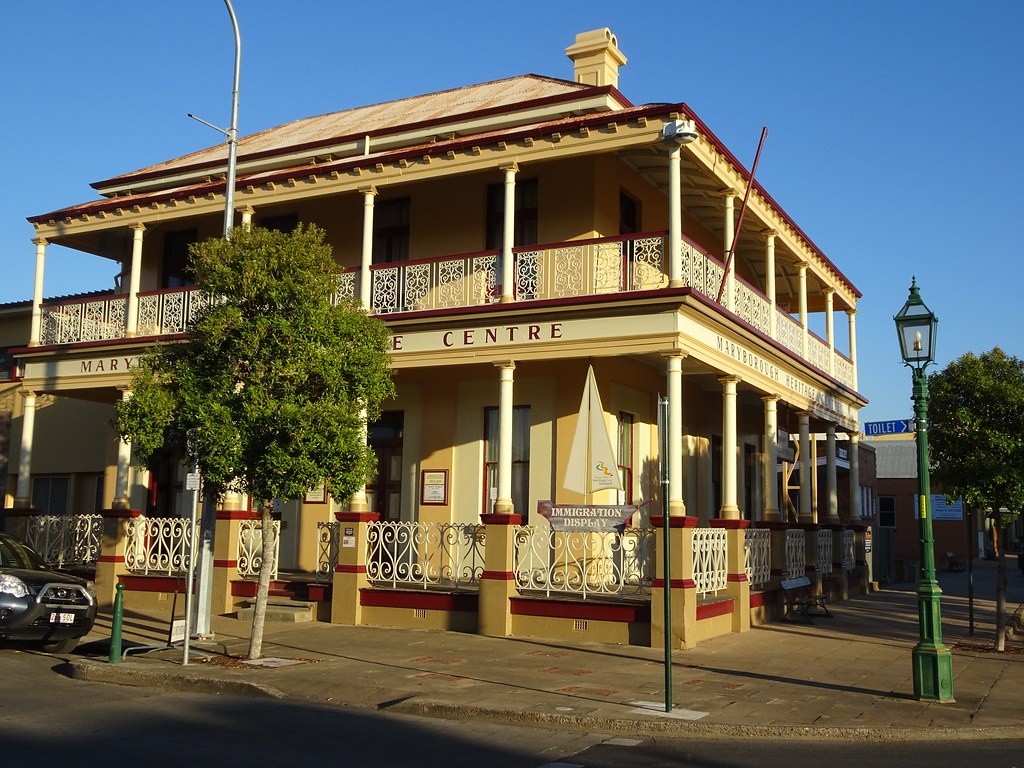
[894,276,957,705]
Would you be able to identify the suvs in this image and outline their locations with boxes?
[0,532,99,655]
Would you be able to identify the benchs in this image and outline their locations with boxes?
[781,575,835,625]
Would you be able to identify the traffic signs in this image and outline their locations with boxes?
[865,418,912,437]
